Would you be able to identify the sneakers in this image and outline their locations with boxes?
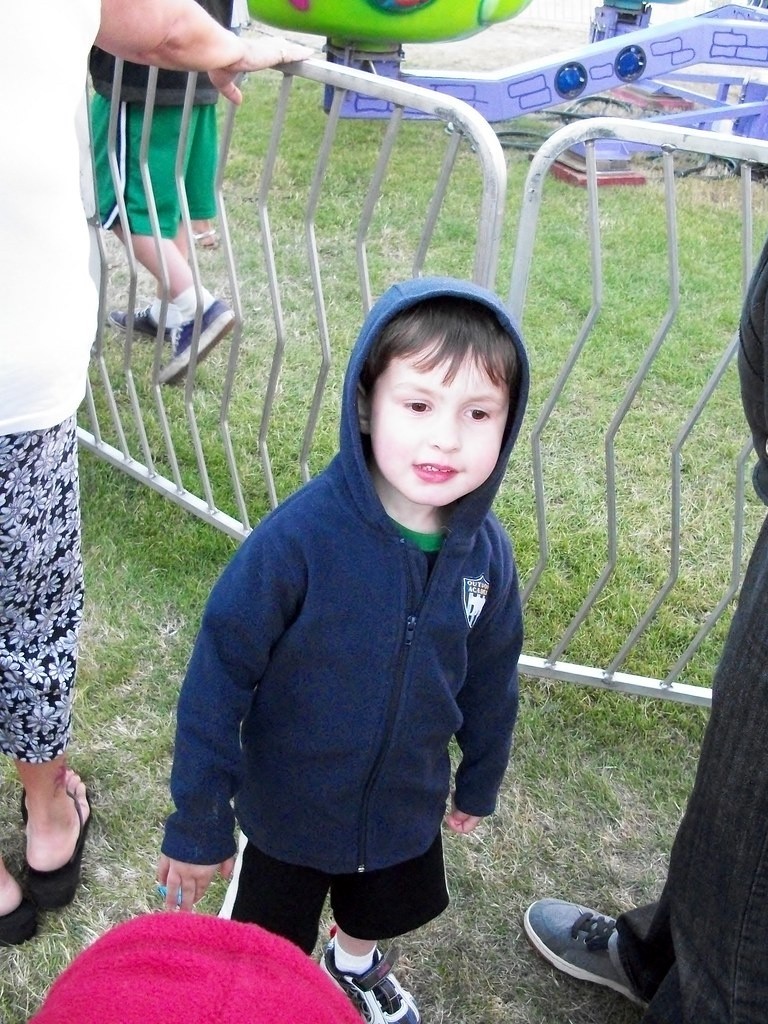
[159,298,237,386]
[108,303,172,337]
[523,897,650,1011]
[319,936,421,1024]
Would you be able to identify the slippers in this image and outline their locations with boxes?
[21,782,92,908]
[0,894,37,944]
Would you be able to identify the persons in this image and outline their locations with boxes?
[0,0,316,947]
[152,274,524,1023]
[522,230,768,1024]
